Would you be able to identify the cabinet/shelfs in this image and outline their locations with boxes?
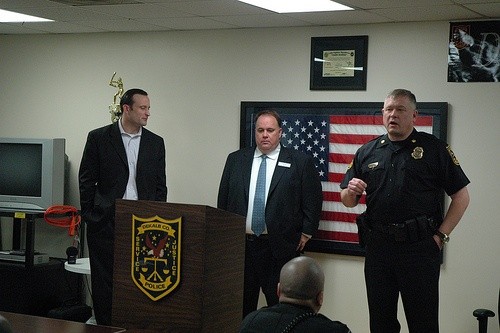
[0,210,92,323]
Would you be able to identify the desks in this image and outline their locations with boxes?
[65,257,97,325]
[0,312,125,333]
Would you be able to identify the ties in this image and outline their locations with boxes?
[251,154,266,237]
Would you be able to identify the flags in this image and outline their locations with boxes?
[251,115,433,244]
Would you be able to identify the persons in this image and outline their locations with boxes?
[340,89,470,333]
[217,111,323,321]
[238,256,351,333]
[79,88,168,326]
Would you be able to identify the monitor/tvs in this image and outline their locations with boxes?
[0,138,68,211]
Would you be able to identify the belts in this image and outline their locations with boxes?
[363,214,441,233]
[245,235,267,241]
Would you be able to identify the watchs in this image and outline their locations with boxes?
[435,230,450,244]
